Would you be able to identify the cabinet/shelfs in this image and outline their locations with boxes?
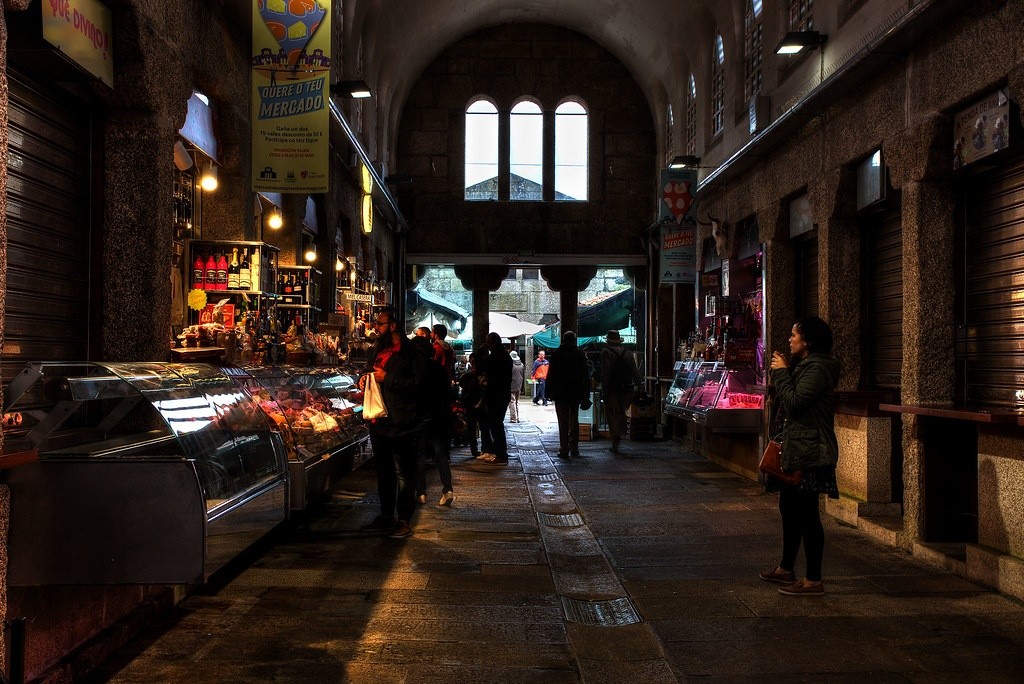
[184,239,280,345]
[277,265,323,332]
[336,262,395,340]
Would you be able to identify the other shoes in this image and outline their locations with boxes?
[533,400,540,405]
[557,451,568,458]
[608,446,618,452]
[417,495,426,504]
[759,566,796,584]
[511,420,516,423]
[476,452,491,459]
[571,451,579,455]
[389,525,412,538]
[484,458,508,465]
[360,519,391,532]
[440,491,453,506]
[484,455,497,461]
[778,577,824,595]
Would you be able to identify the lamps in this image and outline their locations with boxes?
[330,81,373,99]
[267,205,283,229]
[173,141,195,171]
[773,31,828,55]
[668,155,701,169]
[386,174,415,187]
[201,160,218,192]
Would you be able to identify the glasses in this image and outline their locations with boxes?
[374,320,394,326]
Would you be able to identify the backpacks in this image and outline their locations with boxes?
[609,349,632,383]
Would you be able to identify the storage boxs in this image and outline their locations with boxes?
[579,423,591,440]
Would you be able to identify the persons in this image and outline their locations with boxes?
[460,353,493,458]
[530,350,549,406]
[759,316,842,596]
[593,329,646,451]
[356,307,467,539]
[507,350,524,423]
[472,332,513,465]
[545,330,593,460]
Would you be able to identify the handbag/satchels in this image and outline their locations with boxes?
[758,440,802,483]
[363,373,389,419]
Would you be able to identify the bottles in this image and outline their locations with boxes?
[192,246,321,339]
[334,261,395,338]
[676,316,718,359]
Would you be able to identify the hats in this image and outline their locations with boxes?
[509,351,520,361]
[607,329,624,343]
